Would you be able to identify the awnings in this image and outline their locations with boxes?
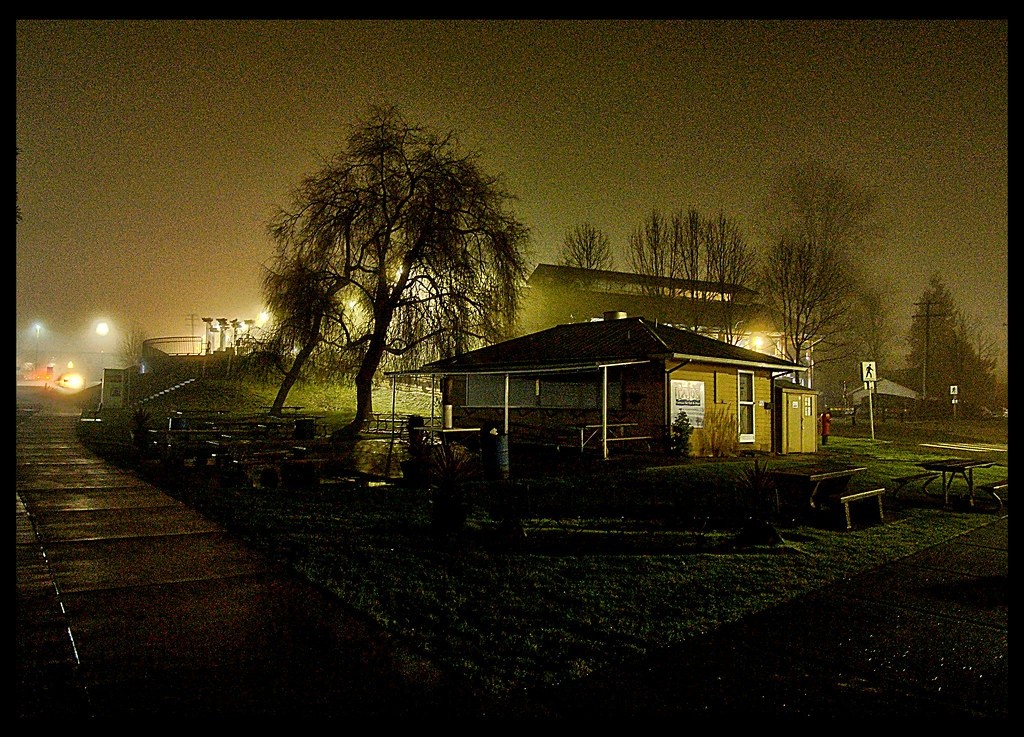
[385,360,651,482]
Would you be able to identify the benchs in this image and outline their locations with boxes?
[547,432,603,456]
[360,418,411,434]
[819,484,887,532]
[889,473,942,500]
[596,435,654,459]
[975,479,1008,515]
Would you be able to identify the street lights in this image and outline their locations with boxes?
[95,322,108,377]
[33,324,43,373]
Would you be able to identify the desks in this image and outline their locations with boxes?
[145,405,332,482]
[412,425,481,461]
[763,461,868,526]
[368,412,401,432]
[564,422,639,456]
[421,416,442,427]
[914,458,997,508]
